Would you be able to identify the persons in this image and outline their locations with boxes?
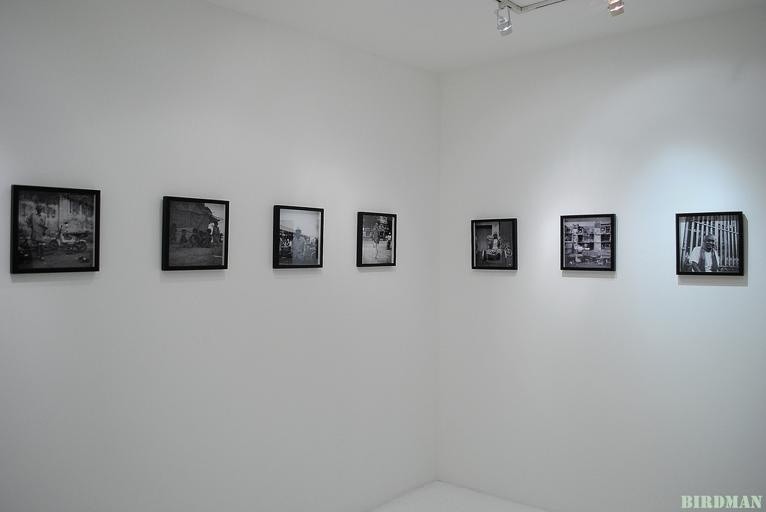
[687,234,723,272]
[290,224,306,261]
[366,222,386,260]
[482,232,509,258]
[26,201,51,260]
[167,217,224,248]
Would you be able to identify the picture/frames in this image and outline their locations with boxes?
[560,214,616,271]
[676,211,744,277]
[471,218,517,270]
[356,212,396,267]
[163,196,229,270]
[272,205,324,268]
[11,184,100,274]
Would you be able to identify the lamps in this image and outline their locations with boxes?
[608,0,625,16]
[497,0,512,35]
